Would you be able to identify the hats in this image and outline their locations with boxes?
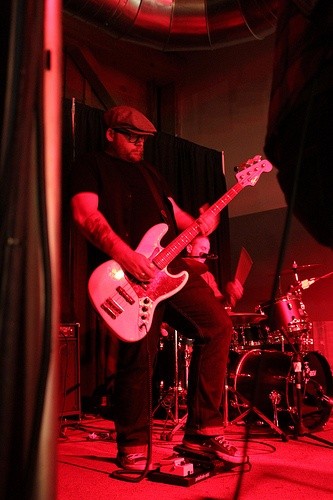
[104,104,159,137]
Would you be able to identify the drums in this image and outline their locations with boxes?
[234,349,333,432]
[255,294,309,343]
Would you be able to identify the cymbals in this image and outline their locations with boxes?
[288,272,333,295]
[283,263,319,272]
[225,307,268,318]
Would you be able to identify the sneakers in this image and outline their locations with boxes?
[182,431,247,464]
[116,453,154,471]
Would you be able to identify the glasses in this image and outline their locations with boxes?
[114,128,147,142]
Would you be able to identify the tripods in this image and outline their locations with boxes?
[151,328,189,438]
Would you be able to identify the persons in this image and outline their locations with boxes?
[157,234,244,388]
[71,104,248,471]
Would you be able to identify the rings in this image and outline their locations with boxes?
[140,273,146,279]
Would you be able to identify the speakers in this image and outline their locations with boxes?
[58,322,82,417]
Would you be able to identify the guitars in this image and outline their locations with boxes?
[88,154,272,341]
